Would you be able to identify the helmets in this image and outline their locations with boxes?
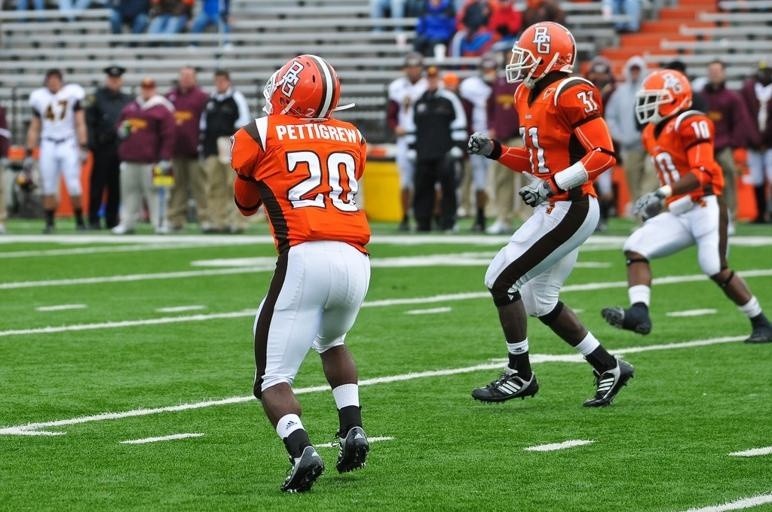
[262,53,341,124]
[400,52,612,83]
[634,68,693,125]
[504,20,578,89]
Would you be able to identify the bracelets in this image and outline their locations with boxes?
[659,185,672,197]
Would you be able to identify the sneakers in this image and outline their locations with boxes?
[397,216,513,235]
[582,357,635,408]
[0,221,249,234]
[281,445,326,494]
[601,305,653,335]
[472,364,540,404]
[743,322,772,344]
[336,426,370,474]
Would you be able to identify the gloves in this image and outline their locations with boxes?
[465,132,495,157]
[632,189,666,222]
[518,171,555,208]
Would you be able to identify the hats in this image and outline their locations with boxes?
[754,58,772,73]
[140,77,156,88]
[101,66,127,77]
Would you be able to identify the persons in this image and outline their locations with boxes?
[602,69,771,343]
[0,0,230,48]
[469,21,635,407]
[229,54,372,494]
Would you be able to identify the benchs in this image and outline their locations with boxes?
[2,0,770,119]
